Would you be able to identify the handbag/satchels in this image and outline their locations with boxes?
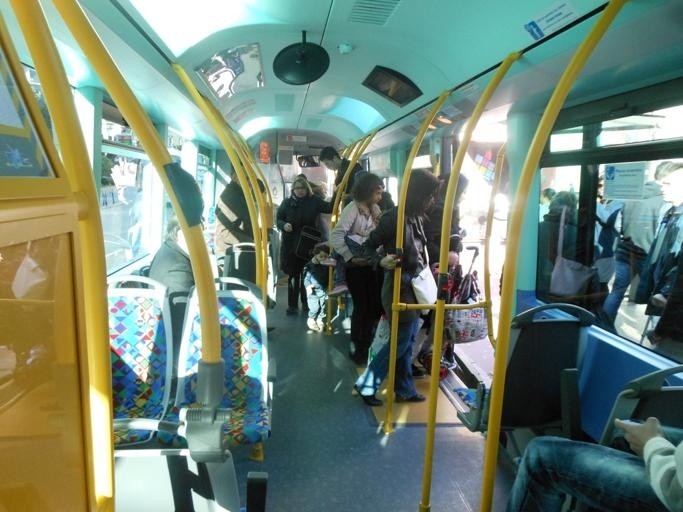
[549,256,601,301]
[295,225,324,262]
[328,234,369,260]
[432,268,453,291]
[446,308,488,343]
[410,266,438,315]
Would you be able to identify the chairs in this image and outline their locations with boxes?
[267,230,279,264]
[223,244,278,304]
[456,303,595,431]
[598,366,683,456]
[174,276,273,444]
[139,265,151,289]
[107,276,174,446]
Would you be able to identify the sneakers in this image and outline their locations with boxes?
[440,356,457,371]
[306,318,320,331]
[316,318,325,328]
[286,307,299,313]
[412,366,426,379]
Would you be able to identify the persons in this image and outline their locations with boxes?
[419,173,469,373]
[300,157,314,167]
[353,168,439,405]
[343,171,394,210]
[543,191,577,222]
[319,147,363,194]
[302,244,330,333]
[596,160,683,324]
[149,212,204,303]
[297,174,324,198]
[539,187,557,222]
[276,177,337,313]
[216,161,254,279]
[330,173,384,360]
[505,415,683,512]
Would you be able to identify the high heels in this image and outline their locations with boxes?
[395,392,431,402]
[351,383,383,407]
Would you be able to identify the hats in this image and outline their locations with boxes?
[293,174,308,189]
